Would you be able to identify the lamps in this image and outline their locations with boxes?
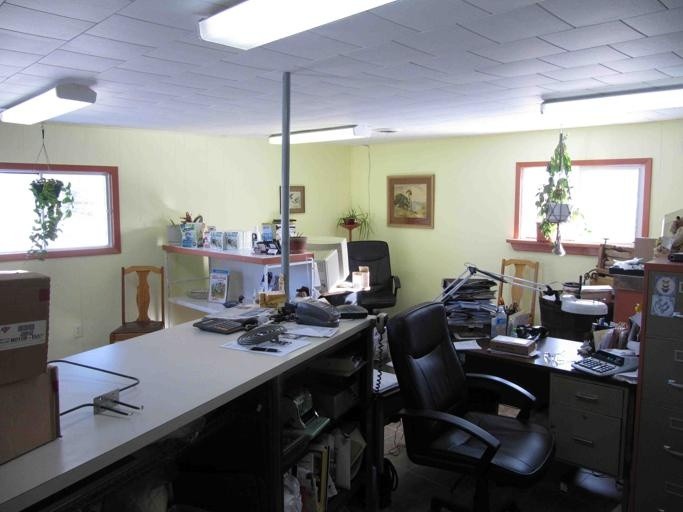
[540,85,681,120]
[265,125,369,147]
[198,1,395,50]
[433,261,610,317]
[0,83,98,126]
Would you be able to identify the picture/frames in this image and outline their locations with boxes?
[384,173,435,230]
[279,185,305,214]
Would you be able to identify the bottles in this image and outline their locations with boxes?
[597,243,606,268]
[495,306,508,336]
[597,318,605,326]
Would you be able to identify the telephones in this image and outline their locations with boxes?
[296,300,340,327]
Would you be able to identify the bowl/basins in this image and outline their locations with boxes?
[563,281,579,294]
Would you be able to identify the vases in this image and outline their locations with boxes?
[335,206,374,239]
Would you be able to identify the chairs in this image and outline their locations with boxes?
[386,302,554,512]
[347,240,401,314]
[108,265,166,344]
[498,257,539,326]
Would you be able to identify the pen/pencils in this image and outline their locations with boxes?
[249,347,282,355]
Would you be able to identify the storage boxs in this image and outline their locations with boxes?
[0,366,61,467]
[0,270,51,385]
[611,272,644,293]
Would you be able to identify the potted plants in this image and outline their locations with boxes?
[536,144,574,244]
[26,178,75,267]
[289,229,308,254]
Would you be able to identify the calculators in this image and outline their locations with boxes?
[193,318,242,335]
[570,349,639,378]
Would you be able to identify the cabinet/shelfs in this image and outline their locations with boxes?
[629,254,682,511]
[162,243,314,329]
[0,314,378,512]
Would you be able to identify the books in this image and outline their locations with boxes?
[439,273,496,337]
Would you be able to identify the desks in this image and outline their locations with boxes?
[371,328,637,512]
